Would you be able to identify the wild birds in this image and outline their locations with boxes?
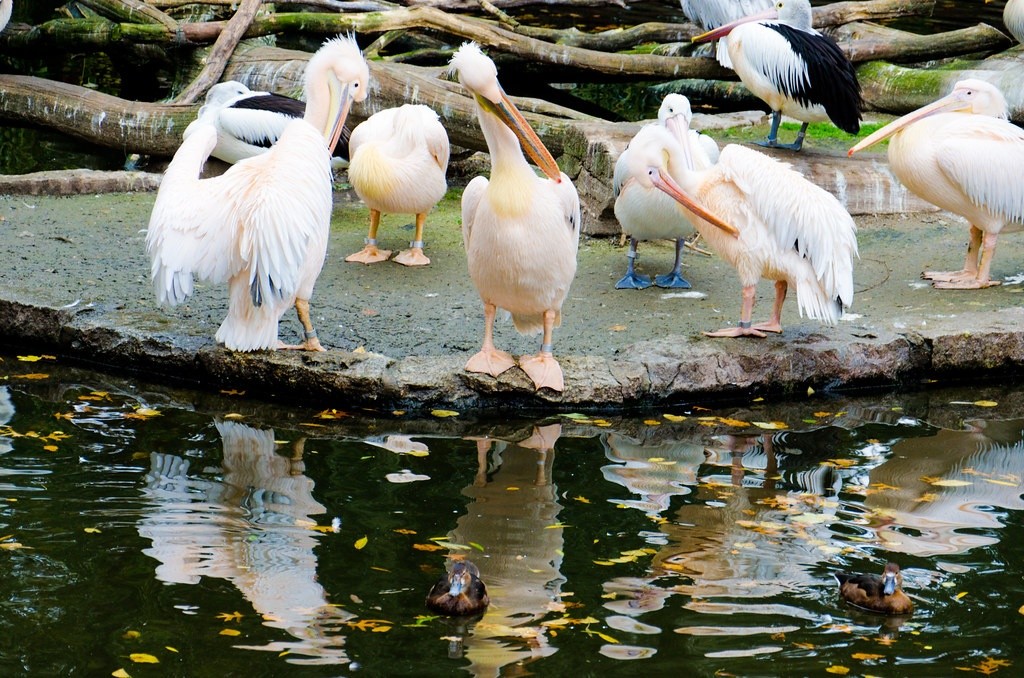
[142,27,370,353]
[848,80,1024,289]
[680,0,863,150]
[612,92,859,339]
[445,42,581,394]
[347,103,451,267]
[136,419,1023,678]
